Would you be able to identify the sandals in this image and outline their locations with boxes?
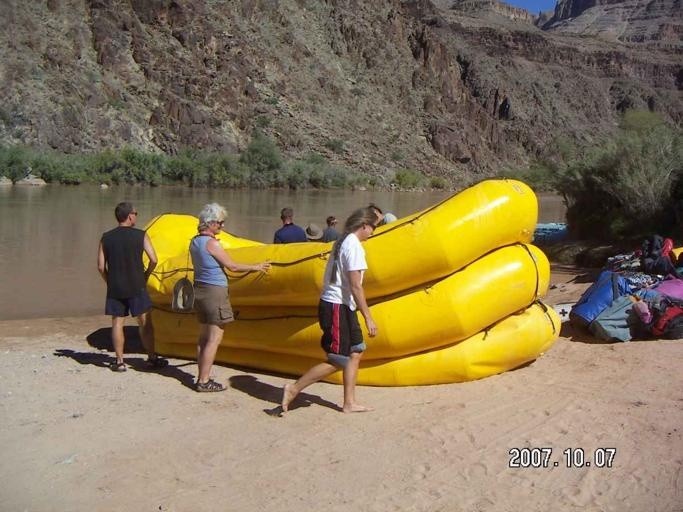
[196,380,226,391]
[147,358,168,367]
[112,360,126,371]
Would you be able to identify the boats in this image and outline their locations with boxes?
[149,245,548,362]
[136,302,562,387]
[535,222,568,244]
[142,179,537,304]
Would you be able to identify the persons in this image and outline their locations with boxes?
[274,204,398,244]
[189,202,270,392]
[282,207,377,412]
[95,200,168,374]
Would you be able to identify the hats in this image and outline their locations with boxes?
[172,278,195,311]
[305,224,323,240]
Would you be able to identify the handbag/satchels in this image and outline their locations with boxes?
[569,270,683,341]
